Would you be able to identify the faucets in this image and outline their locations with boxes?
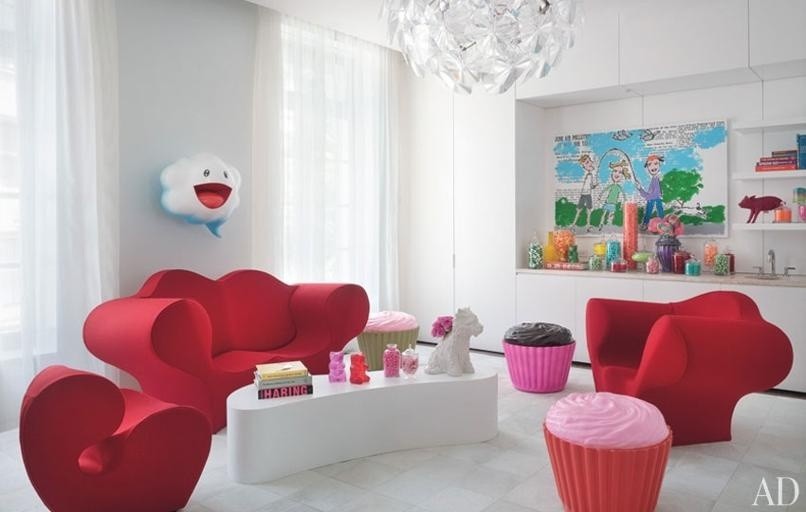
[768,249,777,277]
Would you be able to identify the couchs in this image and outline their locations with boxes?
[586,291,794,448]
[82,269,370,435]
[20,364,212,512]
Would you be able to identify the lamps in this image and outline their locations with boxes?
[378,0,589,101]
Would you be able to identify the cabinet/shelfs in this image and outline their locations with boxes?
[722,280,806,397]
[731,116,806,231]
[515,14,642,117]
[514,269,643,367]
[620,1,764,97]
[395,52,454,346]
[749,0,806,83]
[454,76,552,355]
[643,275,722,305]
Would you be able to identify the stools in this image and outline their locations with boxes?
[502,322,576,394]
[541,391,674,512]
[357,313,420,372]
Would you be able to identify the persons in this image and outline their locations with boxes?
[635,154,665,230]
[328,352,345,383]
[569,154,598,232]
[599,160,630,231]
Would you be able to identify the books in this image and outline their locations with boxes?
[253,360,313,399]
[754,132,806,171]
